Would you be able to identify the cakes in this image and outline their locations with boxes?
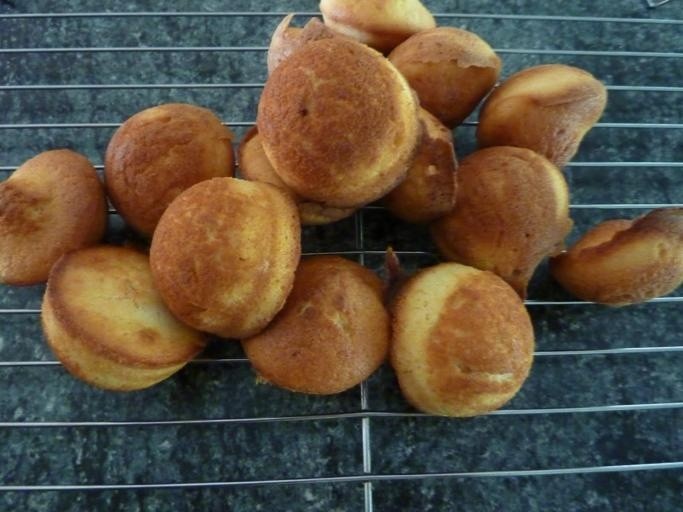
[386,26,502,125]
[1,148,107,285]
[318,1,439,54]
[266,13,343,75]
[428,147,572,297]
[381,108,458,226]
[240,256,391,396]
[550,204,683,309]
[479,64,608,170]
[240,122,355,223]
[391,263,537,418]
[105,104,235,238]
[258,38,421,205]
[148,177,303,338]
[40,247,207,394]
